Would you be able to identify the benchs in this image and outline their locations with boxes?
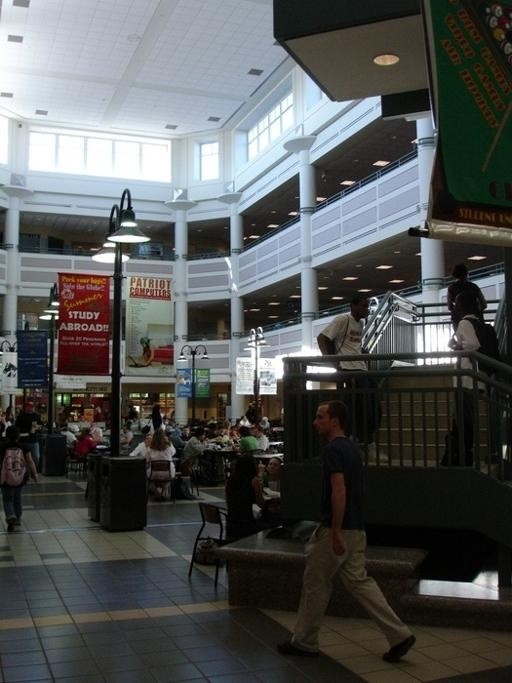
[214,524,428,622]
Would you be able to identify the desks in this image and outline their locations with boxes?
[252,452,284,466]
[218,445,238,479]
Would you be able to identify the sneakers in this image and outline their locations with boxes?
[7,519,16,531]
[16,516,22,525]
[365,442,388,461]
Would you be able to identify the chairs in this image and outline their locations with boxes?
[144,460,175,504]
[68,440,86,477]
[187,502,232,591]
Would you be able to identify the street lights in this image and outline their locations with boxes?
[38,283,69,476]
[178,345,210,420]
[0,340,28,424]
[243,326,271,421]
[91,187,151,531]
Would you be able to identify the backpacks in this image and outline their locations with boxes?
[1,446,25,486]
[457,317,499,379]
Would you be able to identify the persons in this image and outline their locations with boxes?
[119,423,133,448]
[86,422,104,445]
[140,335,159,365]
[74,246,87,257]
[0,402,21,439]
[237,426,259,456]
[71,426,103,461]
[183,428,214,485]
[129,424,151,453]
[0,425,41,532]
[151,405,166,434]
[437,291,493,470]
[446,264,488,332]
[275,399,417,663]
[144,428,176,500]
[255,425,270,454]
[180,426,192,443]
[225,454,280,544]
[16,396,43,486]
[260,456,284,492]
[257,415,272,436]
[166,425,184,458]
[63,425,80,457]
[129,433,153,457]
[317,292,390,464]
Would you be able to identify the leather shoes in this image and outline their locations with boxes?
[382,635,416,661]
[276,634,319,656]
